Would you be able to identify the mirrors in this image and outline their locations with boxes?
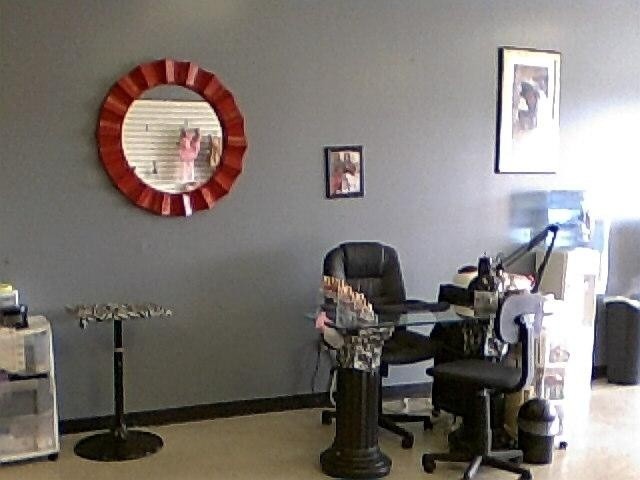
[95,58,247,218]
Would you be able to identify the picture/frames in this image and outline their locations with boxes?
[495,46,562,174]
[325,145,364,199]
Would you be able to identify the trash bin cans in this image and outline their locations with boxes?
[517,397,558,464]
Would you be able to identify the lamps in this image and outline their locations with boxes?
[468,225,559,292]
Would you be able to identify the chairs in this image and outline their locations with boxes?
[422,293,544,480]
[322,242,440,449]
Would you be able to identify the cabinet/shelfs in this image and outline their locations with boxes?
[0,315,60,463]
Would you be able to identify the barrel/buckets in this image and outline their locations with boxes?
[505,190,548,248]
[546,190,586,249]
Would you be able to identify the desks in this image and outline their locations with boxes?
[65,303,173,462]
[305,303,495,480]
